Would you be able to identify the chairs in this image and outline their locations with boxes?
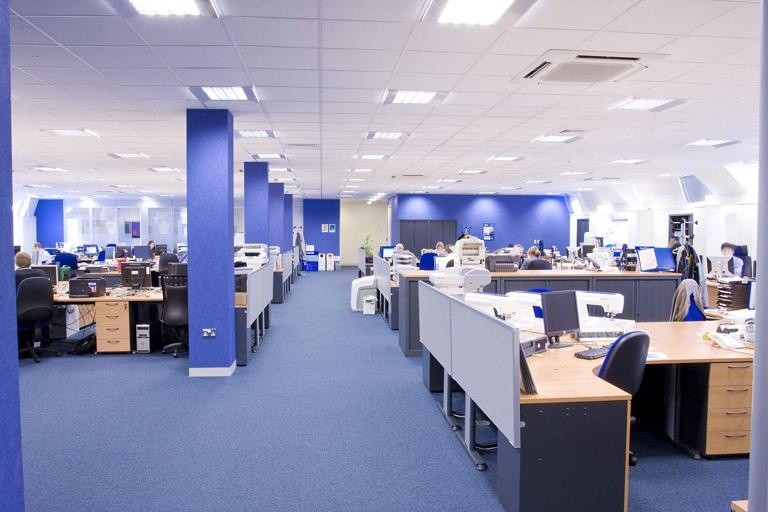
[17,276,62,362]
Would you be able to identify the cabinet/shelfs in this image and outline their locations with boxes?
[301,251,318,271]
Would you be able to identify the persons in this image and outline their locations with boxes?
[148,241,155,253]
[520,246,545,270]
[721,242,744,277]
[34,242,51,265]
[392,242,415,265]
[436,241,444,256]
[15,252,46,286]
[443,245,455,267]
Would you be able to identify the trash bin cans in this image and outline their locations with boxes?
[363,296,376,314]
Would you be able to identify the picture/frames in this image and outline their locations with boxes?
[322,224,328,232]
[329,224,335,232]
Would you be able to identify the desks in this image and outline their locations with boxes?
[357,245,755,512]
[52,245,301,366]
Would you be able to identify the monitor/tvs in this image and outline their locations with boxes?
[121,262,152,296]
[635,246,658,272]
[105,247,115,264]
[541,290,581,348]
[706,256,735,281]
[543,248,554,258]
[580,243,596,257]
[566,247,581,260]
[380,245,396,259]
[117,246,131,258]
[31,266,58,293]
[155,245,168,256]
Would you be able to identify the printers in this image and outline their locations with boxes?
[69,277,106,298]
[454,237,485,271]
[485,254,518,272]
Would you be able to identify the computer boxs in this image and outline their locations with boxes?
[136,314,151,354]
[518,331,549,358]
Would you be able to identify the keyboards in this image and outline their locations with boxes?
[575,346,610,360]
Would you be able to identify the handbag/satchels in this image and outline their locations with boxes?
[76,333,96,355]
